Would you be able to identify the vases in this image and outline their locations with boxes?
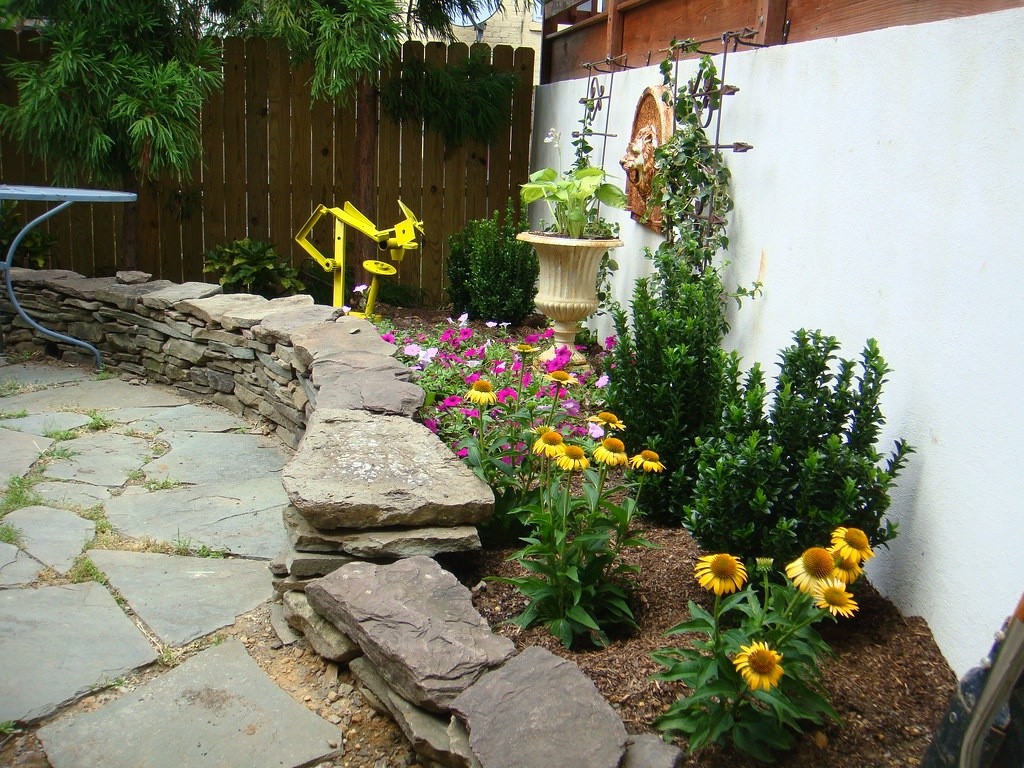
[515,232,624,375]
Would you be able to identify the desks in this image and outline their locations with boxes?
[0,184,137,373]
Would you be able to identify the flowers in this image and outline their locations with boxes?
[340,284,668,652]
[646,527,876,761]
[519,128,628,240]
